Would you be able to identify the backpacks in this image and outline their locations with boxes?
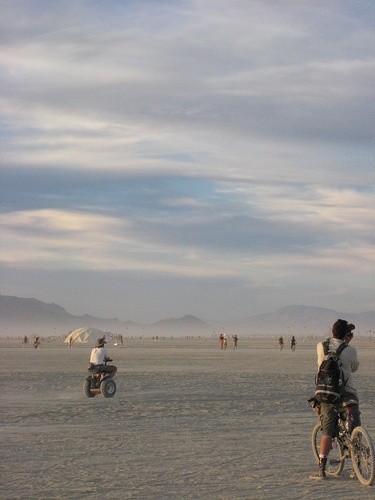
[314,339,350,405]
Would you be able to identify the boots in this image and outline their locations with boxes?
[350,456,362,478]
[309,458,327,479]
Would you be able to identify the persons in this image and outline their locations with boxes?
[316,319,360,477]
[24,335,125,347]
[220,334,298,351]
[88,338,118,380]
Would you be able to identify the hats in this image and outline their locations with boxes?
[95,338,108,347]
[332,319,355,337]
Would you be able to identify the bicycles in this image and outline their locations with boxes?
[307,395,374,486]
